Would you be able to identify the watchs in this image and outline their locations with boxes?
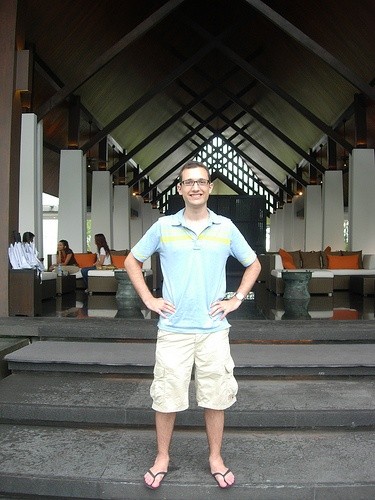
[234,292,246,302]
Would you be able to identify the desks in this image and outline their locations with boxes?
[282,271,312,300]
[115,271,146,306]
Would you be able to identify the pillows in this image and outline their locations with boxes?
[75,253,97,267]
[112,255,125,268]
[279,246,362,269]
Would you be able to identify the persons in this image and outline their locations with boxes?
[81,234,112,293]
[22,232,35,245]
[123,161,261,489]
[49,240,79,297]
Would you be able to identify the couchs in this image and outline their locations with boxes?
[41,254,118,295]
[258,252,270,283]
[269,251,375,297]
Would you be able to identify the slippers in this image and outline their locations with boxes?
[143,467,168,491]
[211,466,235,490]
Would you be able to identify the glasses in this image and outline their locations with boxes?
[181,179,210,187]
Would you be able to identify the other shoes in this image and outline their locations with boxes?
[80,289,89,296]
